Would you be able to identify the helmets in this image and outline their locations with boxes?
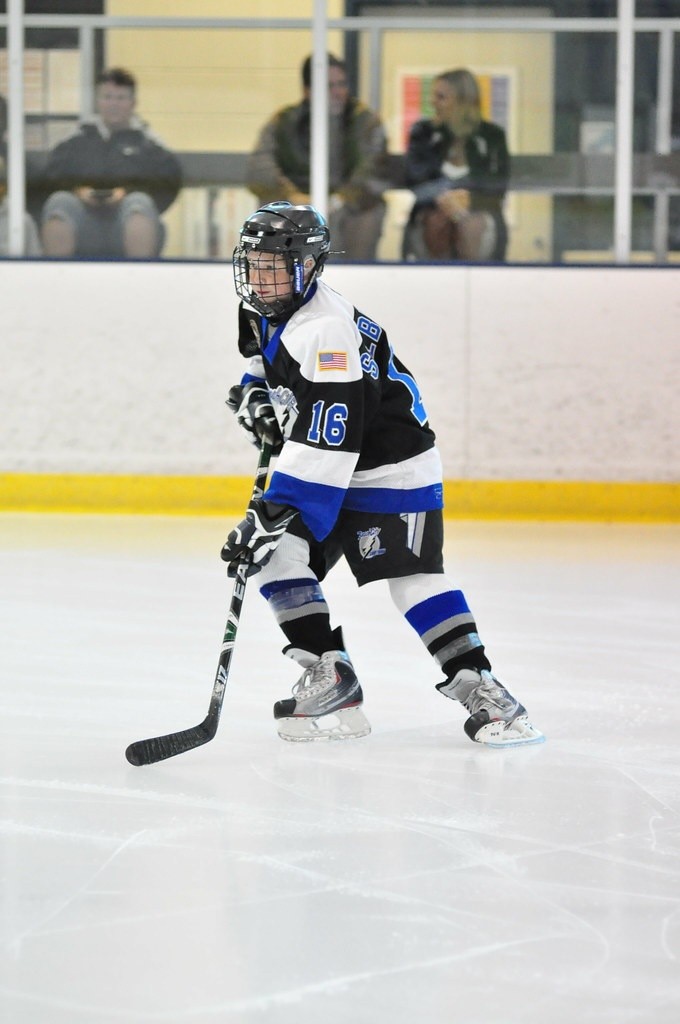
[239,201,330,324]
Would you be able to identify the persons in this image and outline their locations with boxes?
[37,67,182,260]
[0,93,39,258]
[401,69,509,263]
[247,54,388,259]
[221,201,546,748]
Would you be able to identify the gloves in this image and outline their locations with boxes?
[221,500,300,579]
[226,381,283,458]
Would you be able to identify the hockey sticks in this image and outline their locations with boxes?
[124,433,275,768]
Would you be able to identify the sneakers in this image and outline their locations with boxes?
[436,669,544,749]
[273,627,372,743]
